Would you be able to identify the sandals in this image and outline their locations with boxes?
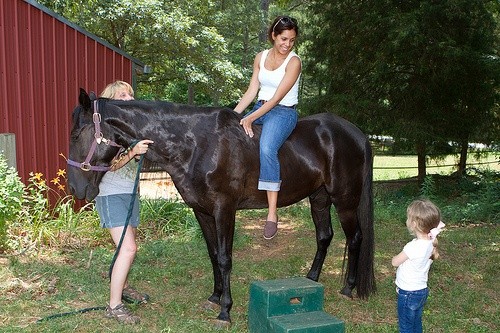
[122,285,149,301]
[104,301,140,325]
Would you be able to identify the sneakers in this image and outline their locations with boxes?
[263,213,279,240]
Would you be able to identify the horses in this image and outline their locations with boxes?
[67,87,377,331]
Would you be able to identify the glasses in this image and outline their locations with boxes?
[273,17,298,32]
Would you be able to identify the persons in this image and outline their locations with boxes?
[233,15,302,240]
[93,81,154,326]
[391,199,441,333]
